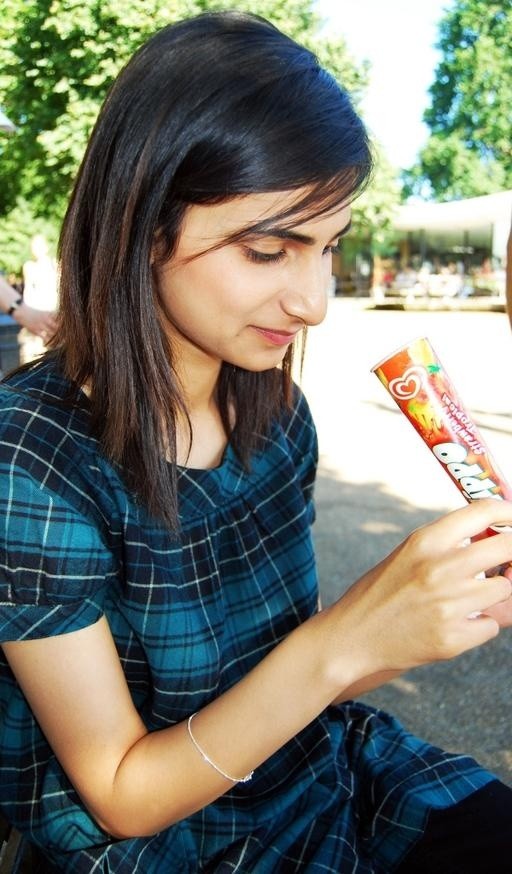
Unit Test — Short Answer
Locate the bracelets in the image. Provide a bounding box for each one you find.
[7,297,24,316]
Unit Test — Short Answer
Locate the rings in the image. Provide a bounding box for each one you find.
[42,330,48,336]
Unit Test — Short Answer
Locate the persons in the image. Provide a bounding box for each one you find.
[0,277,60,378]
[0,9,512,871]
[372,247,506,313]
